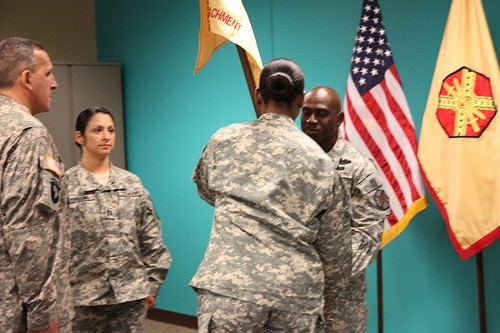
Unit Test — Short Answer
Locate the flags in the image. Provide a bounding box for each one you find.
[335,0,428,256]
[193,0,264,90]
[416,0,500,260]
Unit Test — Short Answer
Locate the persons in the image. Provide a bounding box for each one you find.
[301,86,389,333]
[188,58,336,333]
[66,107,170,333]
[0,36,69,333]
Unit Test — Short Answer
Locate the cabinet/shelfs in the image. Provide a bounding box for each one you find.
[33,60,125,169]
